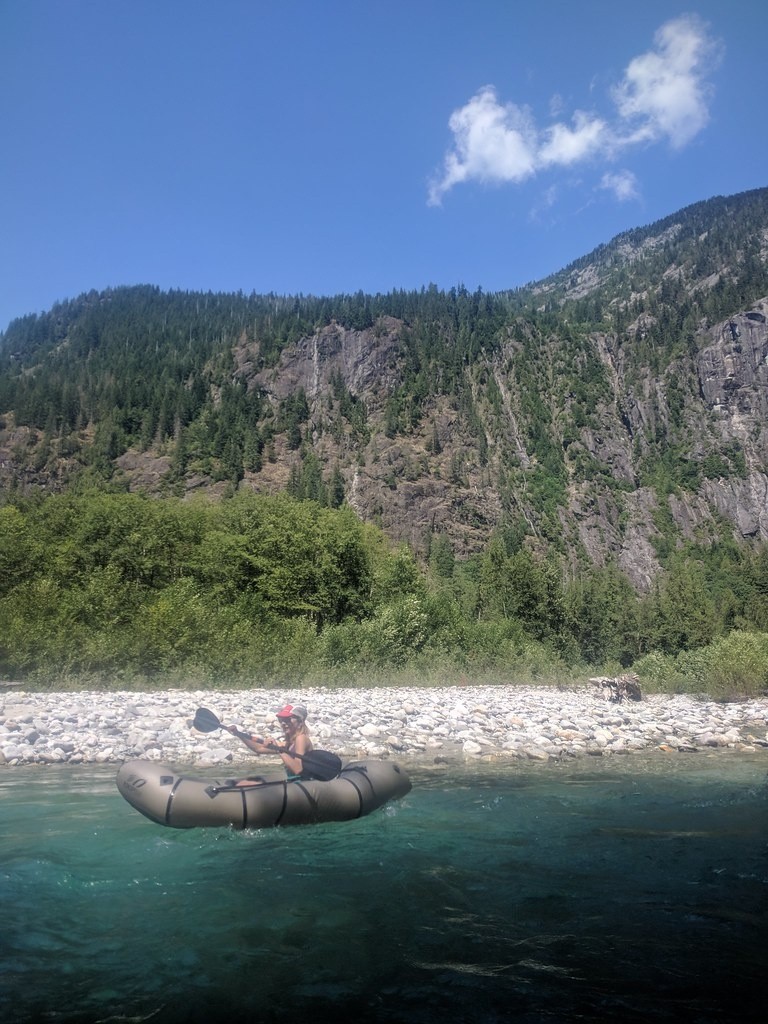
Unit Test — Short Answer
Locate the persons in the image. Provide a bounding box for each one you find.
[228,702,314,786]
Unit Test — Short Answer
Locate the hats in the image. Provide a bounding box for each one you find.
[276,703,308,721]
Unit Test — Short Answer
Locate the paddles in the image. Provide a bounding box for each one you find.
[193,708,342,781]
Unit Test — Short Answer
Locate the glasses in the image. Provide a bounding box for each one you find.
[278,717,291,723]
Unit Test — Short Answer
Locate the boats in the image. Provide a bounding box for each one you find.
[114,761,411,830]
